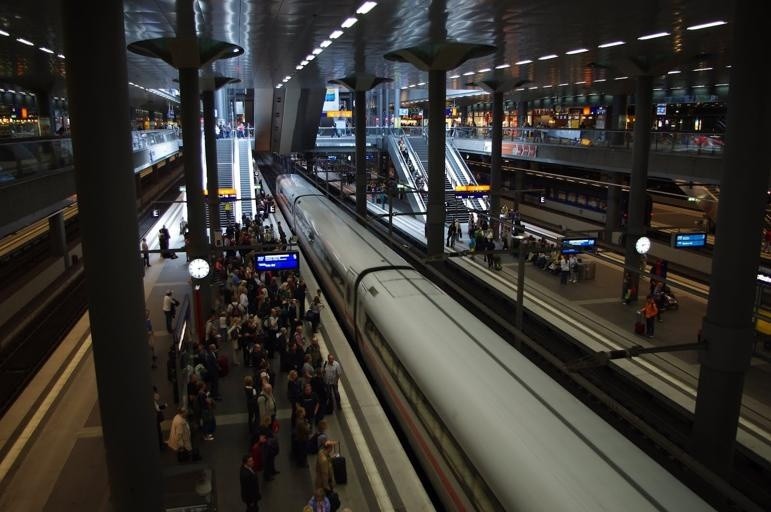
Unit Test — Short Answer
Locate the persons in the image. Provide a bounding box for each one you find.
[316,138,425,207]
[141,193,345,511]
[139,112,248,138]
[620,255,677,339]
[445,201,581,287]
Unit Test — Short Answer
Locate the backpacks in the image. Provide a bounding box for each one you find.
[231,303,241,317]
[307,432,323,454]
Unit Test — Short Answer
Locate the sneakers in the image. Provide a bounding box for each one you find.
[203,434,214,441]
[264,470,279,482]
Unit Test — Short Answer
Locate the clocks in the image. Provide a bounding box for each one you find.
[635,236,651,255]
[187,258,210,279]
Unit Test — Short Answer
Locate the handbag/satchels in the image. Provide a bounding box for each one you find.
[325,490,340,512]
[272,420,280,432]
[306,310,314,321]
[177,447,202,464]
[659,296,670,307]
[191,397,200,410]
[573,264,579,272]
[230,329,238,338]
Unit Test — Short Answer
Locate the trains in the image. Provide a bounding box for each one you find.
[0,127,184,268]
[276,173,771,512]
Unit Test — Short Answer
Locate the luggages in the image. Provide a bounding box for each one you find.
[217,355,230,377]
[331,441,347,484]
[324,385,333,415]
[635,311,645,334]
